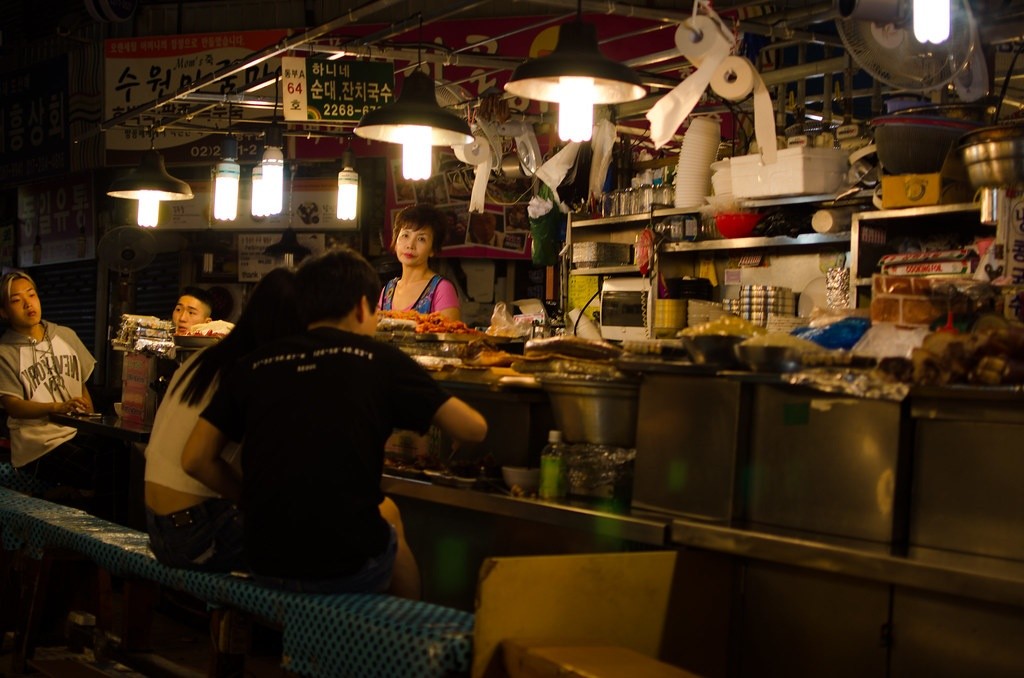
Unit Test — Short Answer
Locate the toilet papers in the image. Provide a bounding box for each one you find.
[645,15,730,150]
[449,136,493,214]
[711,57,778,167]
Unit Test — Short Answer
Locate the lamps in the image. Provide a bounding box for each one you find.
[503,0,647,142]
[353,0,475,180]
[186,163,231,272]
[265,166,311,266]
[106,131,196,229]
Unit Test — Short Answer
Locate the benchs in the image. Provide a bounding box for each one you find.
[0,462,468,678]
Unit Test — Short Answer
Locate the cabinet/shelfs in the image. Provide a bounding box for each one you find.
[562,204,1003,341]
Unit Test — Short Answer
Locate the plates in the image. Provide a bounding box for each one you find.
[171,334,219,348]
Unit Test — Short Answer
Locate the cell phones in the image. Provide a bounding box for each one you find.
[71,411,102,417]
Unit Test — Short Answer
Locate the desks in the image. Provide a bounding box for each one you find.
[49,413,155,525]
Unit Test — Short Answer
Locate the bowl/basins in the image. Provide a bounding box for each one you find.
[957,125,1024,191]
[673,115,732,209]
[715,212,764,239]
[502,465,539,494]
[536,377,641,447]
[687,299,722,327]
[415,333,475,359]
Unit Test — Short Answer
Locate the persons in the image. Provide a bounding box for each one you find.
[144,268,305,574]
[172,286,213,336]
[0,273,97,500]
[378,204,460,322]
[180,251,488,599]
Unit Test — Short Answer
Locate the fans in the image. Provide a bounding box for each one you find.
[97,226,157,286]
[834,0,1001,99]
[434,79,544,177]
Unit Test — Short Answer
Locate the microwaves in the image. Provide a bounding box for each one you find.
[600,276,650,342]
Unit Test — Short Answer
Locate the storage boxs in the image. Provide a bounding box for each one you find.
[120,354,162,421]
[905,374,1024,562]
[752,382,903,543]
[881,142,968,208]
[502,636,702,678]
[869,272,970,330]
[451,385,538,485]
[631,374,745,516]
[724,148,845,201]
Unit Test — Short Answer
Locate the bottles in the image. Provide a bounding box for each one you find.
[601,182,676,217]
[537,428,567,503]
